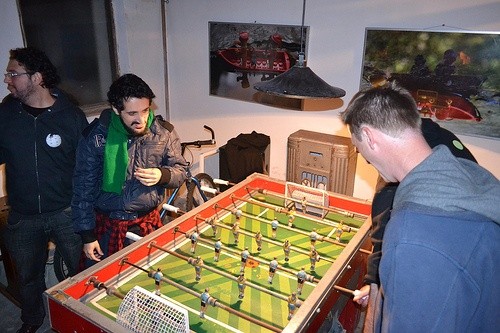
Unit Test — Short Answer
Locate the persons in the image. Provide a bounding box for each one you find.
[343,83,500,333]
[71,73,191,275]
[0,47,90,333]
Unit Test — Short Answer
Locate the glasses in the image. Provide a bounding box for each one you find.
[4,72,29,78]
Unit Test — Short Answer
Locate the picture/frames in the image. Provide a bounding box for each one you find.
[359,27,500,142]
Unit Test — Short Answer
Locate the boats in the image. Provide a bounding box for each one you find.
[406,85,481,121]
[218,48,290,73]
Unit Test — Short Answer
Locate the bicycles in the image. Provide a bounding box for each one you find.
[52,123,218,284]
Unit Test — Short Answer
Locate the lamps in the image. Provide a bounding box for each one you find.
[253,0,348,99]
